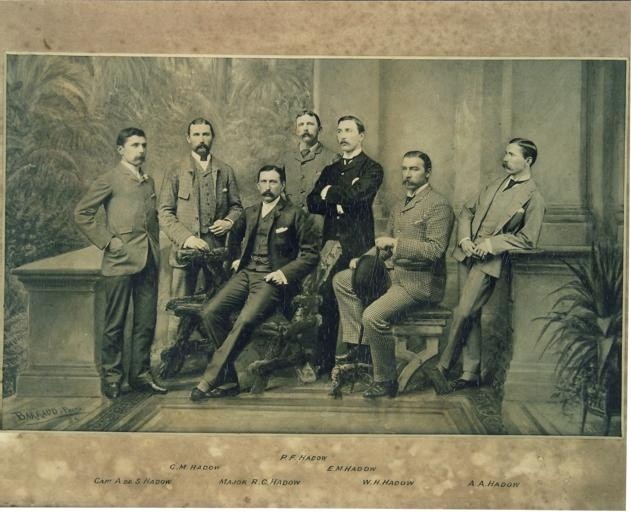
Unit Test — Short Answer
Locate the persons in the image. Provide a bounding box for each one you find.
[331,146,456,398]
[75,126,171,397]
[279,105,334,257]
[305,116,383,379]
[157,109,244,382]
[192,164,323,406]
[432,136,551,392]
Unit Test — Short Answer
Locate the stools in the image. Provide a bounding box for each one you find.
[389,300,453,392]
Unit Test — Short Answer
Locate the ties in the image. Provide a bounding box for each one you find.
[301,148,311,158]
[405,196,413,206]
[344,157,353,165]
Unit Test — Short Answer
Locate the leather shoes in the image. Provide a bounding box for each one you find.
[365,379,400,398]
[103,382,121,399]
[139,383,168,395]
[449,377,473,389]
[190,384,240,401]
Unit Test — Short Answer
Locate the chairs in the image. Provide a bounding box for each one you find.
[162,239,323,395]
